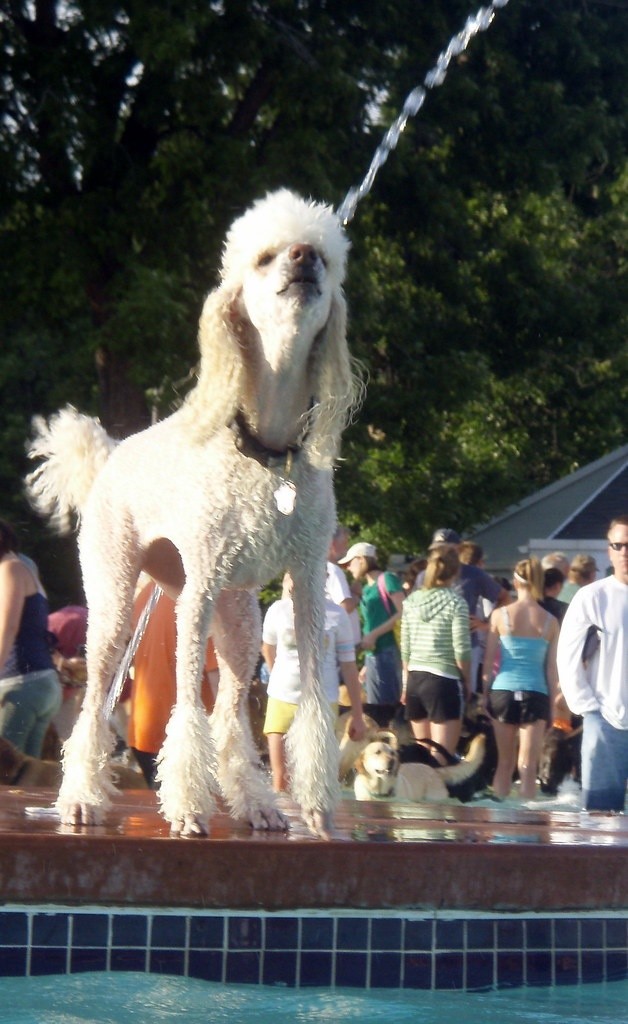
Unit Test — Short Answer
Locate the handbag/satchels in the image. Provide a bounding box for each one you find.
[378,571,401,653]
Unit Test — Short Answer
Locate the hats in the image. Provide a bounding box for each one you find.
[337,541,380,564]
[431,528,464,544]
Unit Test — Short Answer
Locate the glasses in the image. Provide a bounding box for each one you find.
[609,543,628,551]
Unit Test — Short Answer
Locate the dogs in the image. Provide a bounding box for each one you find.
[26,183,373,840]
[337,708,485,807]
[0,737,146,789]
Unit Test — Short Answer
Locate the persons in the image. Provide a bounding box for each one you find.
[0,522,598,804]
[556,511,628,811]
